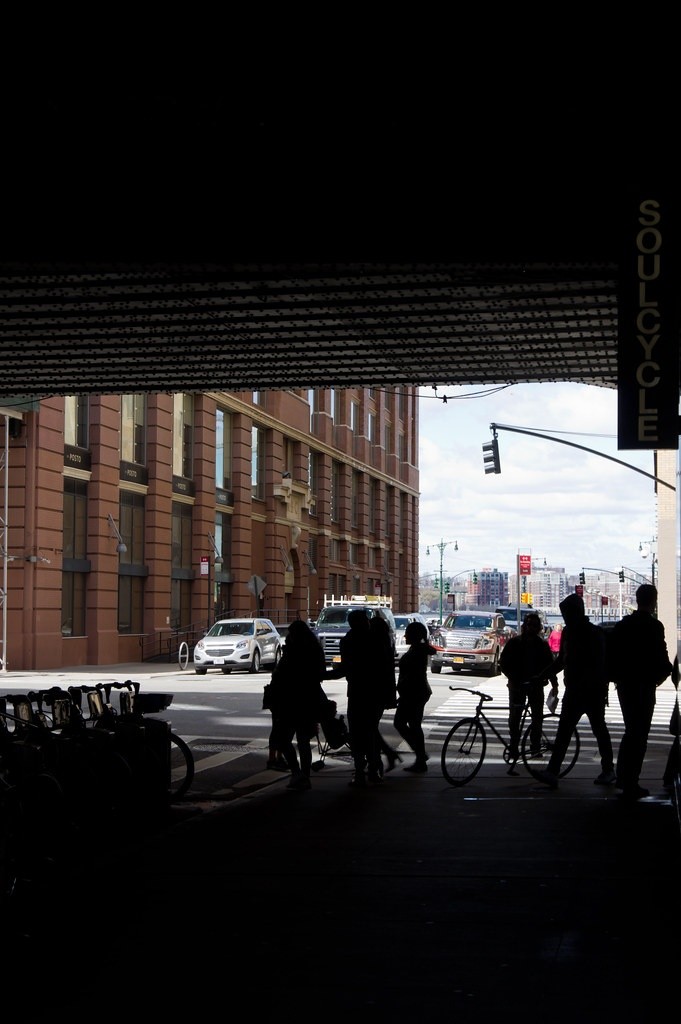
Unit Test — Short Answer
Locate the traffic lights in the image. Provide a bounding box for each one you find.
[579,572,585,584]
[619,571,624,583]
[521,593,532,604]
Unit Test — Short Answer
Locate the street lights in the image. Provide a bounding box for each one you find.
[517,558,547,634]
[638,541,654,585]
[426,540,459,627]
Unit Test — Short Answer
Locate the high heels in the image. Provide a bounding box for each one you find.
[367,760,384,777]
[385,752,403,773]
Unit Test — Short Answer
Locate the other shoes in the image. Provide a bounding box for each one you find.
[404,753,429,774]
[267,757,292,772]
[661,774,675,790]
[530,768,559,788]
[615,780,650,795]
[594,772,618,784]
[531,749,543,758]
[508,748,520,758]
[289,769,312,793]
[367,773,385,785]
[347,772,368,788]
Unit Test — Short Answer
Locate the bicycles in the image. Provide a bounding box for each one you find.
[441,685,580,786]
[0,681,195,909]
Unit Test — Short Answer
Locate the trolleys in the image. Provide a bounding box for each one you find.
[312,708,404,785]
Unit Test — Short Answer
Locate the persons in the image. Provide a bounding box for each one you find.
[531,592,617,788]
[663,657,681,787]
[394,621,436,773]
[549,623,563,660]
[606,584,674,796]
[265,610,396,791]
[500,614,558,758]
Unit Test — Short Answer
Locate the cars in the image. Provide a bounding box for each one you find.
[194,618,283,675]
[308,606,396,673]
[274,624,291,648]
[430,611,516,677]
[496,606,566,637]
[394,612,430,666]
